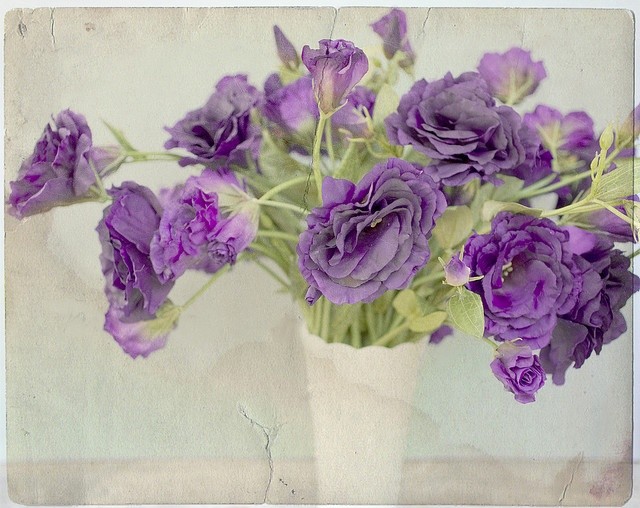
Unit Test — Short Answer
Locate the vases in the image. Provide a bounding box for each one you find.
[299,318,431,503]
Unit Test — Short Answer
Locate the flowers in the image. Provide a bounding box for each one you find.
[4,9,640,404]
